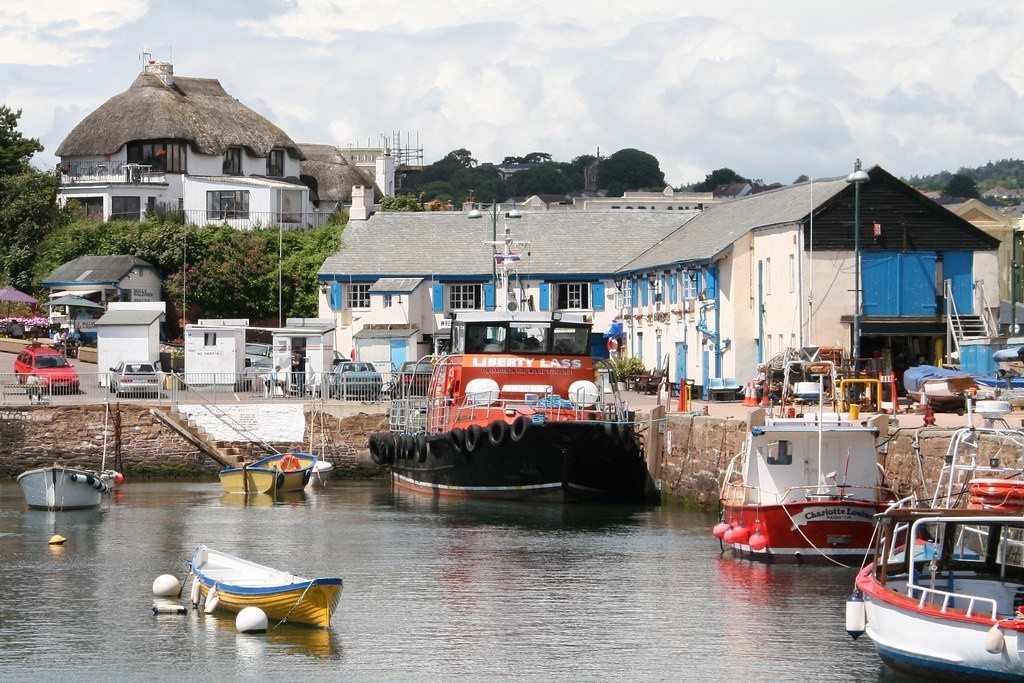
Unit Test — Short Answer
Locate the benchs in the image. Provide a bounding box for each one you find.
[199,561,239,579]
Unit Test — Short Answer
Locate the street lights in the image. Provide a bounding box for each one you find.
[847,158,871,406]
[469,199,522,300]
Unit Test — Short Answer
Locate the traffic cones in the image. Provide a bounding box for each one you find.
[918,384,929,410]
[885,372,906,414]
[747,381,758,406]
[761,380,770,405]
[741,382,750,405]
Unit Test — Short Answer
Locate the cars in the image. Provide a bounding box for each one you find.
[390,361,431,400]
[330,361,382,398]
[109,361,159,396]
[14,347,80,394]
[246,343,273,368]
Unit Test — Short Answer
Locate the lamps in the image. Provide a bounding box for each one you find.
[648,271,659,287]
[688,268,697,283]
[615,276,624,291]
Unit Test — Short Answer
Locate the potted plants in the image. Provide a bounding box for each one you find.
[699,290,706,301]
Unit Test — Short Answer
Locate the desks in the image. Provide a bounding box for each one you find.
[627,374,653,395]
[60,338,75,358]
[24,342,44,348]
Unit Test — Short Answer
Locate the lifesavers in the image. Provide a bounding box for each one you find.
[511,415,533,443]
[449,427,465,454]
[488,419,509,445]
[386,434,414,462]
[280,454,300,473]
[465,423,482,452]
[414,436,427,464]
[966,477,1024,513]
[608,335,622,355]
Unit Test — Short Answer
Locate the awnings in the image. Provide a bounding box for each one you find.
[49,290,101,297]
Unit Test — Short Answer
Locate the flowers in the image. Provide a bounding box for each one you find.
[685,307,695,313]
[635,314,642,319]
[647,312,652,323]
[660,312,669,321]
[671,309,682,314]
[624,314,631,319]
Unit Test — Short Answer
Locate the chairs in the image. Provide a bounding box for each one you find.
[707,377,741,403]
[48,344,79,358]
[627,367,665,395]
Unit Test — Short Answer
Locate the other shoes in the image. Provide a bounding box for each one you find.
[37,402,42,405]
[29,402,33,405]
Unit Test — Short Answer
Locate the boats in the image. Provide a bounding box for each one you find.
[368,225,660,504]
[308,462,333,487]
[17,467,105,509]
[904,367,981,403]
[190,543,342,629]
[712,361,911,565]
[219,453,317,495]
[845,394,1024,683]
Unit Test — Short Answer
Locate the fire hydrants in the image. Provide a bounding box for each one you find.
[922,404,936,427]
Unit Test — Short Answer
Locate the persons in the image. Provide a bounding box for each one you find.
[292,350,305,397]
[8,320,23,339]
[390,371,398,401]
[53,327,88,347]
[270,365,290,398]
[76,308,90,319]
[30,324,42,338]
[27,374,41,405]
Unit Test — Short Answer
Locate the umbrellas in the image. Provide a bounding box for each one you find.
[0,285,38,318]
[42,295,106,329]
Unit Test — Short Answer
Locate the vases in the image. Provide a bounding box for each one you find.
[665,316,669,321]
[677,313,681,319]
[628,318,631,323]
[638,320,641,324]
[688,312,695,318]
